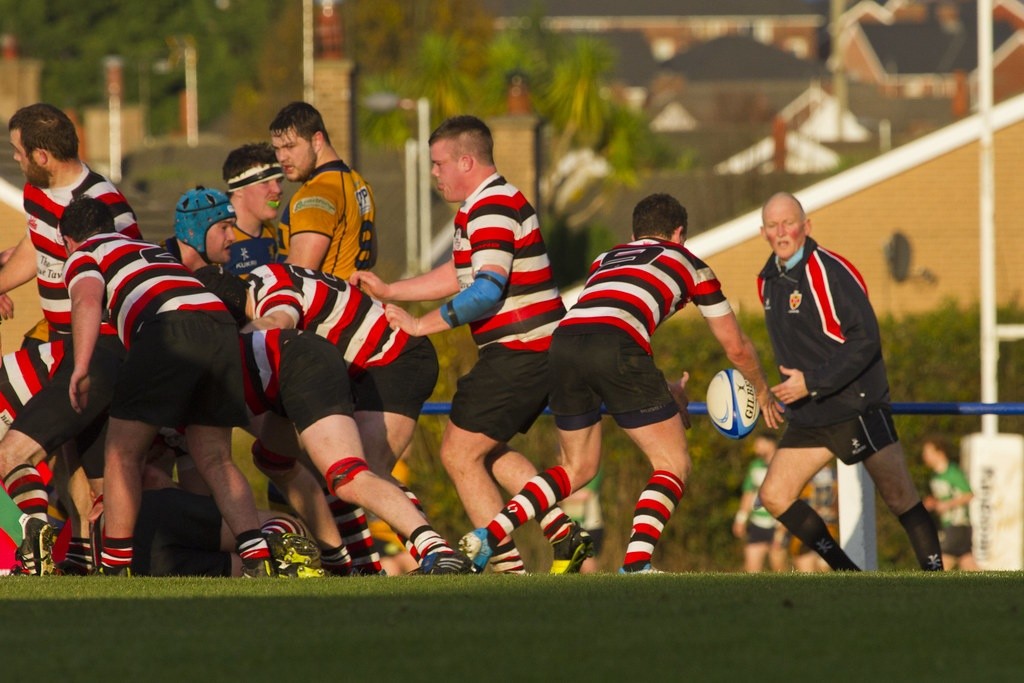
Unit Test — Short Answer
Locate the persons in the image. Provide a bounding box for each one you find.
[558,429,978,574]
[0,102,471,579]
[460,192,785,575]
[757,192,945,573]
[350,114,591,576]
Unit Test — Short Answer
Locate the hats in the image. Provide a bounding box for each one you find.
[173,184,239,266]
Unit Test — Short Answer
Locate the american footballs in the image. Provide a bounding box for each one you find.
[705,364,761,441]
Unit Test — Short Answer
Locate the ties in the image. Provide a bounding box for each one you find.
[772,266,791,318]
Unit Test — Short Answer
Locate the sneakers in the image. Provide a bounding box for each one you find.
[420,550,472,575]
[51,561,87,578]
[459,529,492,574]
[14,517,57,577]
[90,565,133,578]
[546,520,595,575]
[238,557,276,578]
